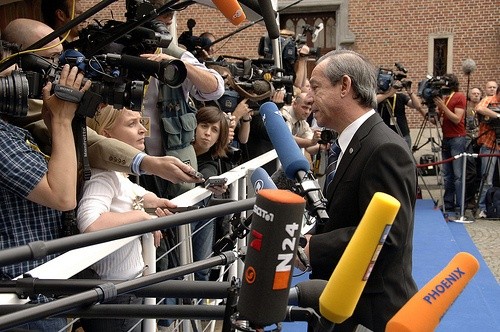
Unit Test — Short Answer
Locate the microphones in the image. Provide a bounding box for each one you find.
[211,0,339,332]
[462,59,476,74]
[385,252,480,332]
[316,192,401,332]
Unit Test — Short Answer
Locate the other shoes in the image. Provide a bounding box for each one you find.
[439,204,455,211]
[455,208,461,215]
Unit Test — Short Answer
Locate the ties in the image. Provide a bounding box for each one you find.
[326,139,341,194]
[292,121,300,135]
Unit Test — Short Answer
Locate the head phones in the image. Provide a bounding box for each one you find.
[468,93,483,101]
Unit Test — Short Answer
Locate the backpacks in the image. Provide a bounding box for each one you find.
[486,187,500,218]
[420,154,440,176]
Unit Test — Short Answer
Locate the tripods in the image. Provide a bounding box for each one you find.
[412,108,448,218]
[473,132,500,217]
[381,99,437,207]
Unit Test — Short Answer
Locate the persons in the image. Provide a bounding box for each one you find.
[418,73,466,216]
[465,88,482,210]
[376,78,421,150]
[475,85,500,220]
[196,31,218,63]
[485,81,497,96]
[293,51,420,332]
[0,19,331,332]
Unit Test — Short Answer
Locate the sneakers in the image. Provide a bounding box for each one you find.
[479,210,487,219]
